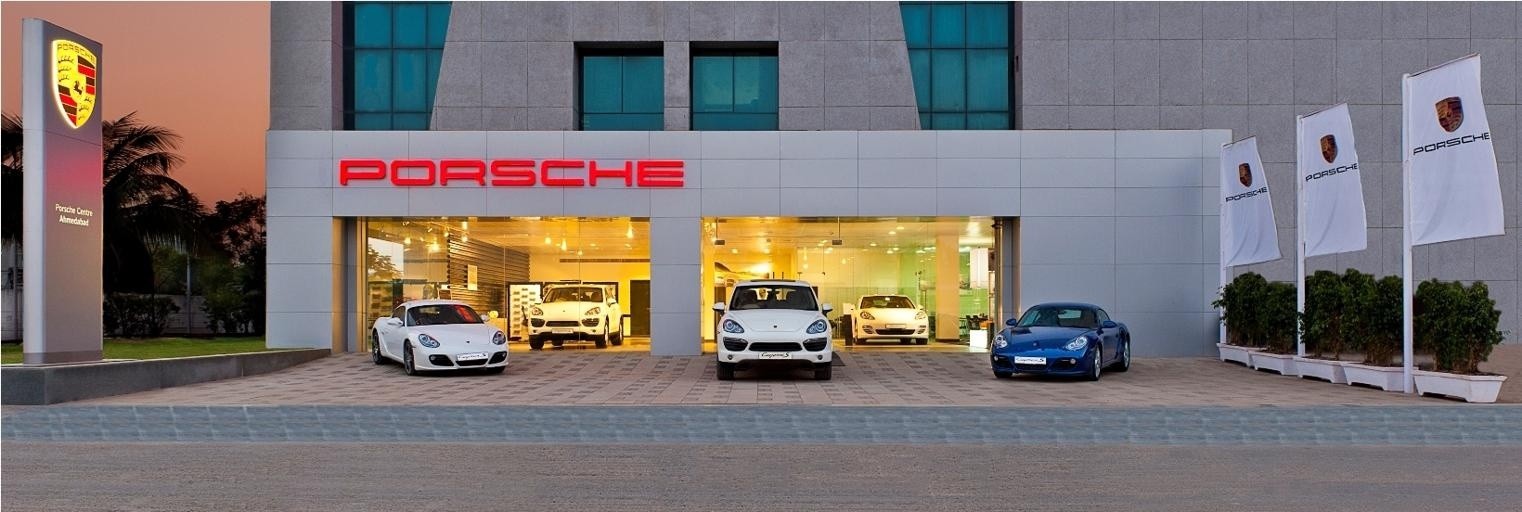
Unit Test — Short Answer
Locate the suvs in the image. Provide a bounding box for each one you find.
[714,279,836,381]
[526,285,626,350]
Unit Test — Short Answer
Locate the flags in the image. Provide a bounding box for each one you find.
[1298,103,1370,257]
[1407,55,1505,247]
[1222,136,1282,270]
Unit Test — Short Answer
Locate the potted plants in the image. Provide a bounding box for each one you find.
[1410,273,1514,404]
[1211,270,1267,368]
[1337,264,1421,397]
[1291,263,1349,387]
[1248,277,1303,378]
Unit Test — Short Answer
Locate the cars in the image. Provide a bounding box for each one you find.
[371,298,509,378]
[991,302,1133,382]
[839,293,931,348]
